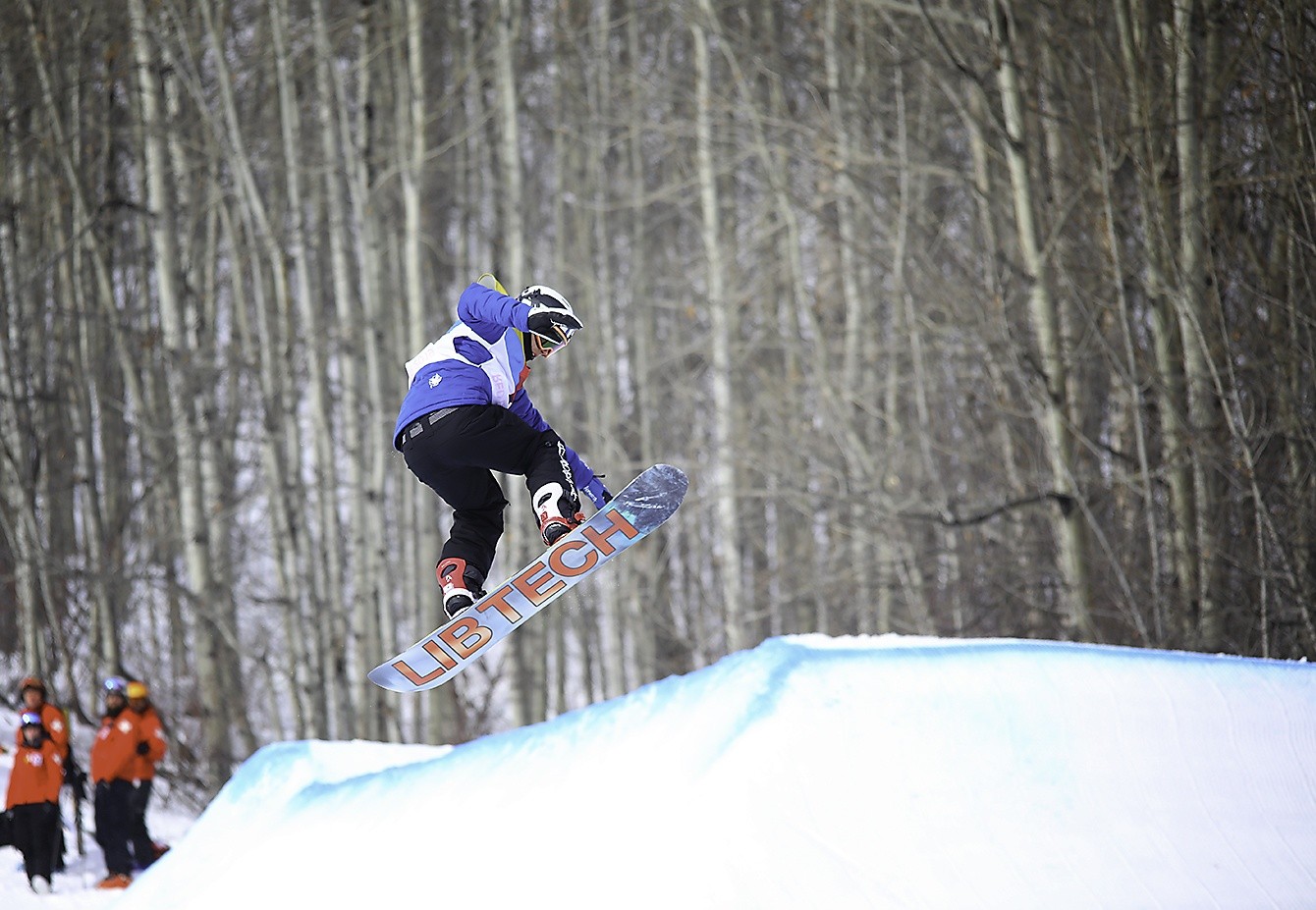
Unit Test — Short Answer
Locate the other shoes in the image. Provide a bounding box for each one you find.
[435,558,484,619]
[532,483,581,547]
[98,872,131,888]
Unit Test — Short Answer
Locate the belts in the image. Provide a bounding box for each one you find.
[402,405,459,445]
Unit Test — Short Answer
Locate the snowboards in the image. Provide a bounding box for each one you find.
[367,463,688,693]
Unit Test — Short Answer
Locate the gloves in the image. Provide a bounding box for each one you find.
[139,739,151,756]
[528,306,583,343]
[583,476,614,511]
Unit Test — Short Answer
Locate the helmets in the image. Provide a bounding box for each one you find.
[19,713,40,728]
[515,285,576,342]
[103,676,128,695]
[127,684,146,699]
[20,677,46,698]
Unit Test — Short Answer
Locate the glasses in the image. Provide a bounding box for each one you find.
[536,325,568,358]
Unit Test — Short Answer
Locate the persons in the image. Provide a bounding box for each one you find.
[90,675,171,888]
[393,274,613,623]
[7,677,69,893]
